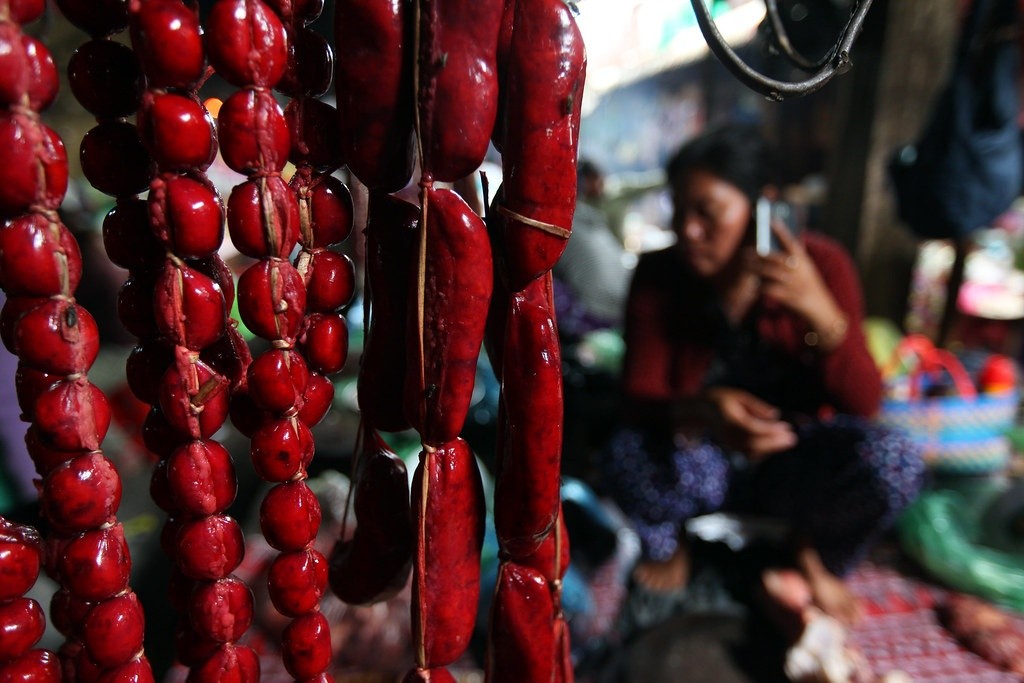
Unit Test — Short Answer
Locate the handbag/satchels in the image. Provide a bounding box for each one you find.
[885,53,1024,241]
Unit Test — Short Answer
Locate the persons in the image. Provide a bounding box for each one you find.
[561,126,924,640]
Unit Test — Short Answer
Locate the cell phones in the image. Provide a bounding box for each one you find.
[757,196,797,258]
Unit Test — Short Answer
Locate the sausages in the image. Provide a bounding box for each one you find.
[0,0,587,683]
